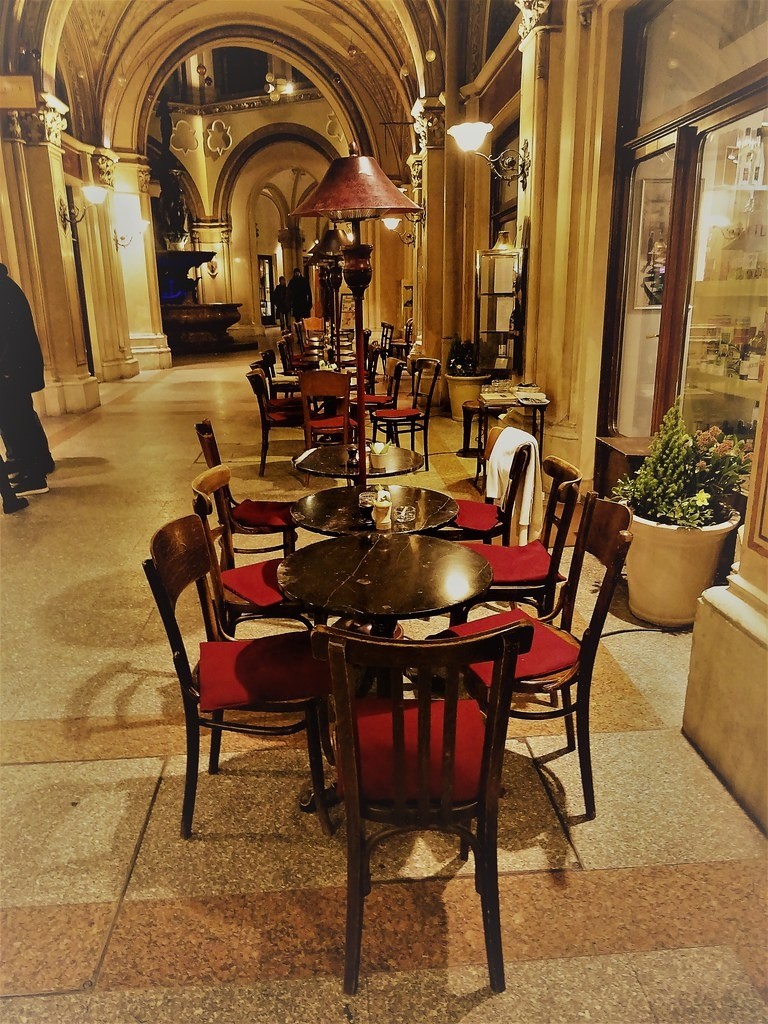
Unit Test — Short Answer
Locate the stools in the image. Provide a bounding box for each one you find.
[461,398,495,457]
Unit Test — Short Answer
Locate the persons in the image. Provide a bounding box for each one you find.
[273,276,291,335]
[0,263,55,497]
[288,268,313,322]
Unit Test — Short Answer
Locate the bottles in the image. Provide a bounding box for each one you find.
[735,126,764,183]
[709,418,758,458]
[699,315,767,382]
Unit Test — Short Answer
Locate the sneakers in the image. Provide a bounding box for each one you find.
[11,472,28,483]
[12,479,50,496]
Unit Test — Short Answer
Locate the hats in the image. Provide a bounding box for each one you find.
[294,268,301,272]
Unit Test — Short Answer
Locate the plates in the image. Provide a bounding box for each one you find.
[517,398,550,405]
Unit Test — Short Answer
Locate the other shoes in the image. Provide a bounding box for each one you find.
[282,332,285,336]
[5,497,29,513]
[5,452,13,460]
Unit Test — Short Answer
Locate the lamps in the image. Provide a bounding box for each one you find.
[59,184,107,232]
[114,218,150,252]
[446,122,530,192]
[382,216,414,248]
[399,186,427,229]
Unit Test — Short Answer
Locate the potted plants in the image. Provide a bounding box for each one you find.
[443,338,492,423]
[624,397,748,630]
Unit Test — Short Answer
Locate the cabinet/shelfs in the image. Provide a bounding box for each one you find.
[685,182,768,403]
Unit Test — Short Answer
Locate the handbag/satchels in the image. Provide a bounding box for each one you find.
[276,307,280,319]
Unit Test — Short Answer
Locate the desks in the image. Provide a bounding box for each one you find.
[304,349,354,355]
[299,355,357,366]
[292,377,370,410]
[310,337,349,341]
[474,395,549,496]
[312,331,350,338]
[291,439,424,482]
[276,534,493,815]
[308,342,353,350]
[295,367,364,376]
[290,485,459,535]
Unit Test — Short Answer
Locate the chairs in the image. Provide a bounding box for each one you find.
[143,320,632,998]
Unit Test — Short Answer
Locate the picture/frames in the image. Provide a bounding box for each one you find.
[632,178,705,311]
